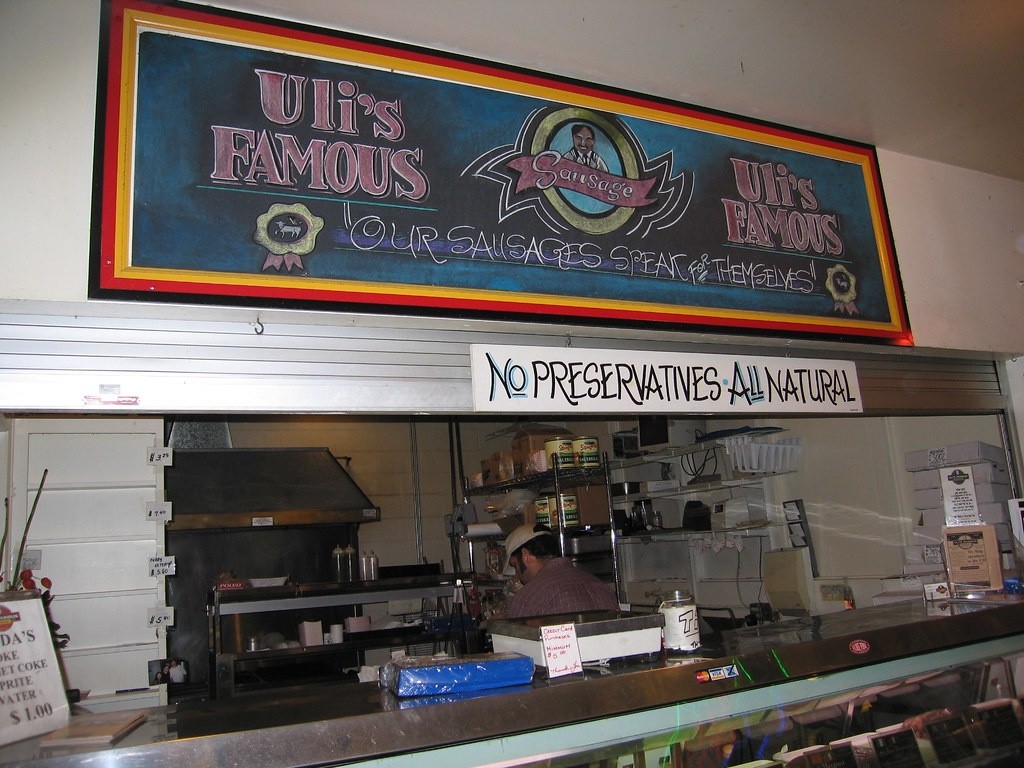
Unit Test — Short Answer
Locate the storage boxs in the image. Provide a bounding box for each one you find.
[511,426,577,464]
[640,479,680,492]
[871,440,1013,606]
[378,650,536,697]
[480,459,499,485]
[490,452,515,482]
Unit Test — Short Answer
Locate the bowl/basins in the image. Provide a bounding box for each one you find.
[250,577,288,587]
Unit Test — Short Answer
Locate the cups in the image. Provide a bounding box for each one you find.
[330,624,344,643]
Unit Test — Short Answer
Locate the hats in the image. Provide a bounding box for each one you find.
[502,522,553,576]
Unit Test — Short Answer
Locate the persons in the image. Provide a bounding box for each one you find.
[501,524,616,620]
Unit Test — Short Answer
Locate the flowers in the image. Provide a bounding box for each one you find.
[0,569,70,649]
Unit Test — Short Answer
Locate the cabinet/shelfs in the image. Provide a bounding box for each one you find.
[464,451,622,601]
[608,437,777,608]
[207,570,476,701]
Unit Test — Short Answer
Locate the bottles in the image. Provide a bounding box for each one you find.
[485,541,502,576]
[369,550,379,581]
[248,636,259,651]
[483,589,506,614]
[660,589,702,658]
[842,576,855,610]
[358,551,370,581]
[344,544,357,583]
[331,544,345,583]
[653,510,663,530]
[634,499,653,530]
